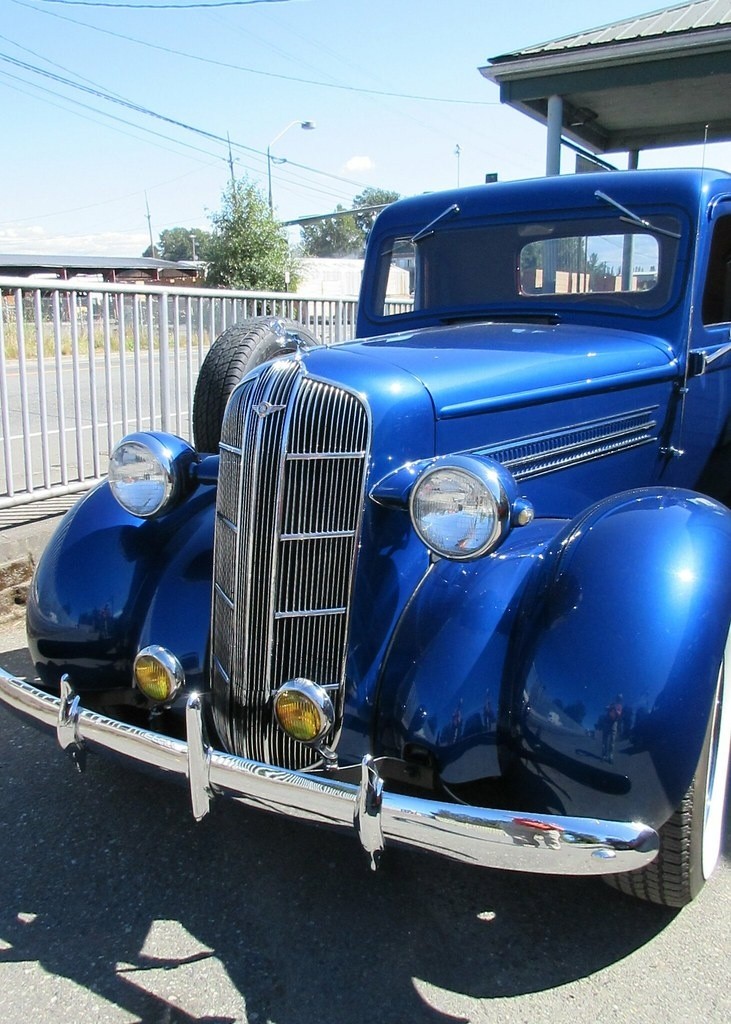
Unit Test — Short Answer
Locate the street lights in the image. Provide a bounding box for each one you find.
[267,120,318,258]
[454,142,461,189]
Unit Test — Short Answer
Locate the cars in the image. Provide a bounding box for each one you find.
[0,165,730,909]
